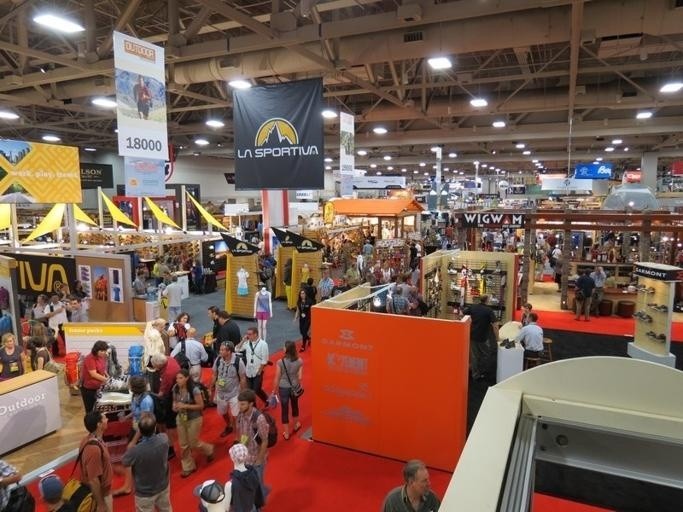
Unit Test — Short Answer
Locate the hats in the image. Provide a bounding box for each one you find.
[194,479,225,504]
[37,474,63,498]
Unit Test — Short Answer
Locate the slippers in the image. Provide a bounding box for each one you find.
[294,423,302,432]
[283,431,290,440]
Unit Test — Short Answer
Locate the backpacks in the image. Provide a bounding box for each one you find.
[252,411,277,448]
[192,382,212,407]
[150,392,166,425]
[174,341,189,369]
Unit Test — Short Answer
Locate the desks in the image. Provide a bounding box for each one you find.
[0,369,61,458]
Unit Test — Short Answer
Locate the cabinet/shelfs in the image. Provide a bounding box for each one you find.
[633,286,669,344]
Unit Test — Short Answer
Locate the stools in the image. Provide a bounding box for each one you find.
[540,338,553,362]
[524,357,541,370]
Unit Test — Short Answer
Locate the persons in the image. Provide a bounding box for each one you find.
[317,196,611,380]
[0,196,311,512]
[381,459,441,511]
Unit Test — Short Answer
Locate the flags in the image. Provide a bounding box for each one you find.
[111,30,170,161]
[228,77,325,192]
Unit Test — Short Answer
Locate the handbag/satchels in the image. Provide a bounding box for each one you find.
[290,385,304,400]
[7,487,35,511]
[200,345,215,368]
[62,479,96,511]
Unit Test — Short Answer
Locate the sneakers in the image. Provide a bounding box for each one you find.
[181,465,197,477]
[208,446,216,463]
[221,426,232,437]
